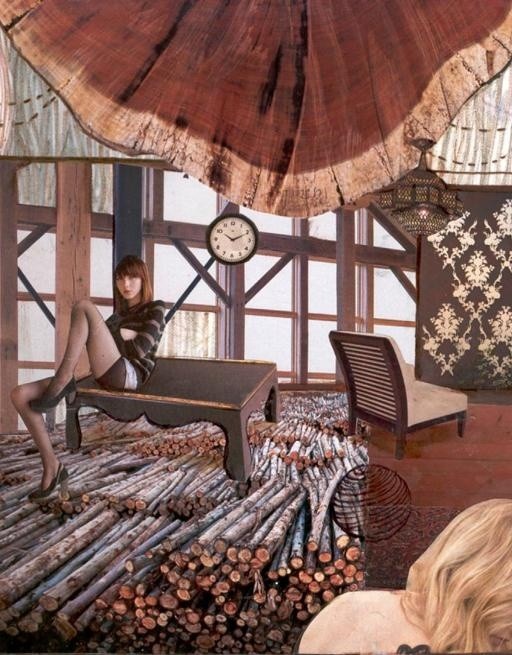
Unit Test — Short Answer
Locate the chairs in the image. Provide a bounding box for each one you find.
[329,333,467,455]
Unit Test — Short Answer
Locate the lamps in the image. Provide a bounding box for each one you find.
[379,138,464,240]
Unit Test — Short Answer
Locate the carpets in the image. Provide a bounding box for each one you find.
[361,505,465,591]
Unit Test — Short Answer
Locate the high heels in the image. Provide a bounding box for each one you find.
[28,463,71,503]
[30,373,79,412]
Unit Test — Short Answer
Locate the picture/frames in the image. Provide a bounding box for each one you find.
[418,186,512,390]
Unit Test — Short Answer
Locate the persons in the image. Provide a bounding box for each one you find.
[400,497,511,654]
[9,256,166,508]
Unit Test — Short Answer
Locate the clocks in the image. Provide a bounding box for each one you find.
[206,213,260,265]
